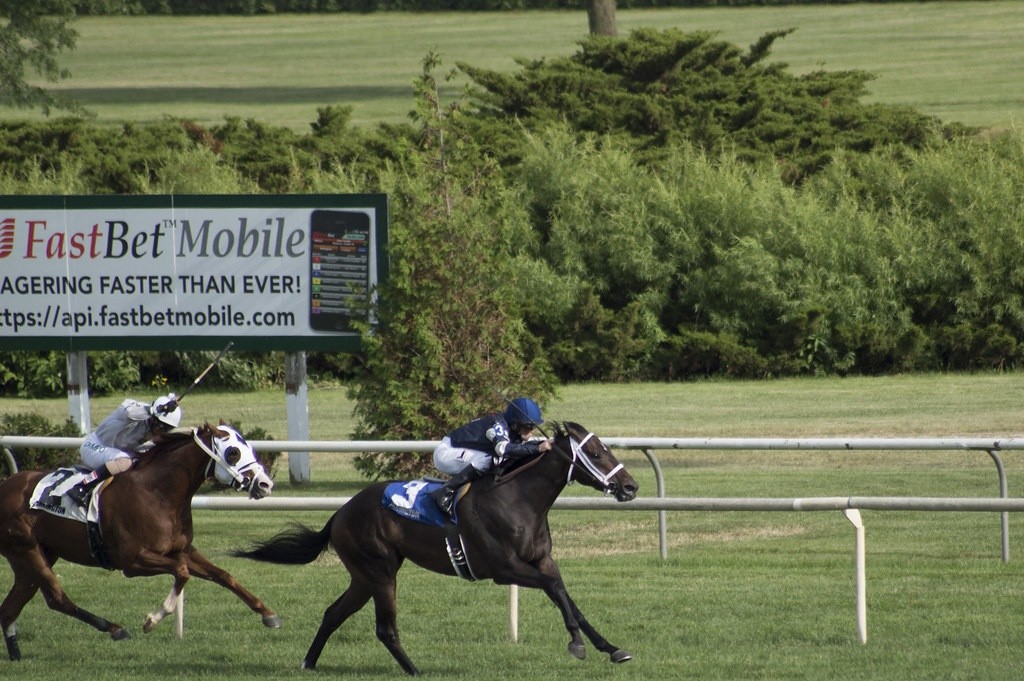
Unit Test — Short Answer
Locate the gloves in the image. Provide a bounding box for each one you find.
[158,400,179,413]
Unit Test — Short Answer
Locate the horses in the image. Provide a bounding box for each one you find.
[221,420,639,675]
[0,419,280,663]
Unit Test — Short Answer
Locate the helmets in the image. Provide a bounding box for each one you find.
[505,398,543,424]
[152,393,181,428]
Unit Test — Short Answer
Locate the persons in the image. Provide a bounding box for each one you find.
[431,397,553,518]
[66,397,182,507]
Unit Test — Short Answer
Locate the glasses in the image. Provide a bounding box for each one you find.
[517,424,536,430]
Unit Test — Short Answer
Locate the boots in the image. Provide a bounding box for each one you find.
[67,463,112,507]
[431,463,481,518]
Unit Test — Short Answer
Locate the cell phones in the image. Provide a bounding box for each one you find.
[308,211,371,333]
